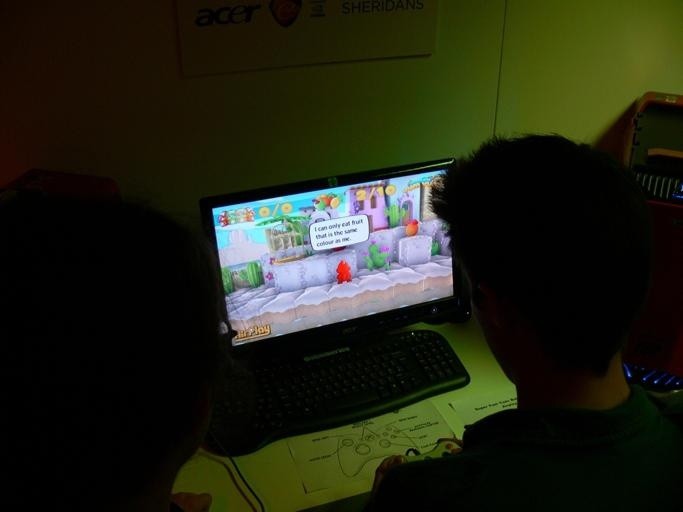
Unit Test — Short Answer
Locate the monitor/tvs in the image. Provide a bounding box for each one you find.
[198,157,466,360]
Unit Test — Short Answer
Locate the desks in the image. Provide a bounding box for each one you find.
[170,297,522,512]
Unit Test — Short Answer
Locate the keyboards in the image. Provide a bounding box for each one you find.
[195,330,471,458]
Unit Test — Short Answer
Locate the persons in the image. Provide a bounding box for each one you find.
[0,188,234,511]
[360,134,683,511]
